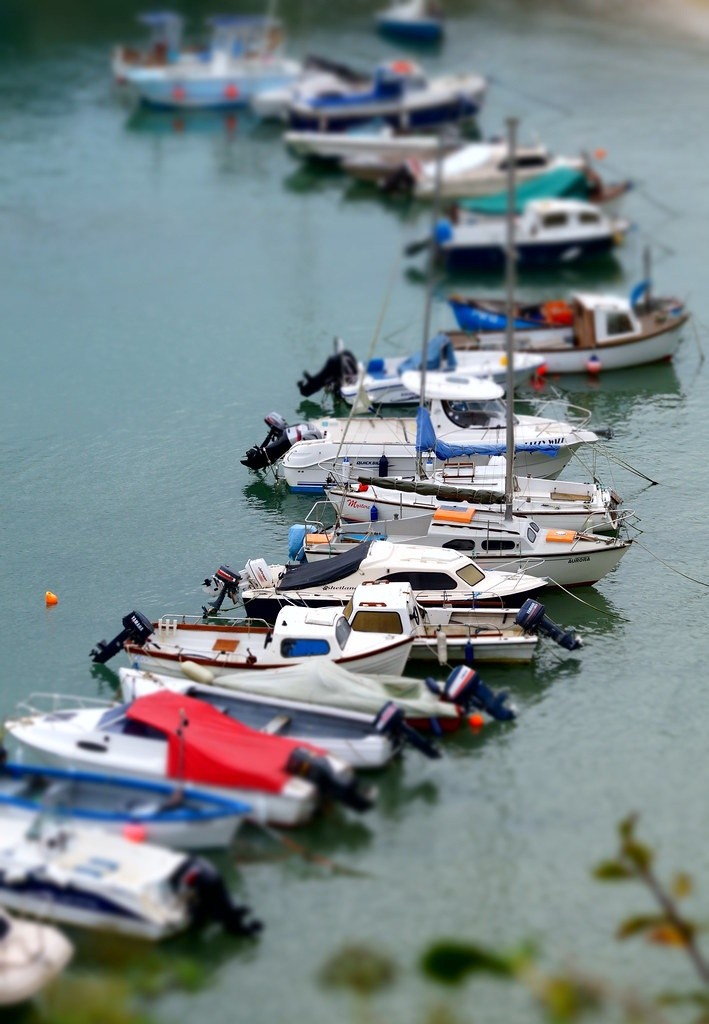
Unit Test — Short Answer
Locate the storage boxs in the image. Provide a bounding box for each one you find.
[245,558,272,588]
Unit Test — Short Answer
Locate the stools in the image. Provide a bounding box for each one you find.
[211,640,239,654]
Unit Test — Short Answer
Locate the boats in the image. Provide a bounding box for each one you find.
[1,285,694,1024]
[456,164,602,221]
[430,195,635,273]
[375,1,447,56]
[246,45,489,131]
[108,10,306,115]
[413,141,632,206]
[278,124,472,191]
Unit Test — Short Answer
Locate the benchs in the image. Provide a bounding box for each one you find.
[551,492,591,502]
